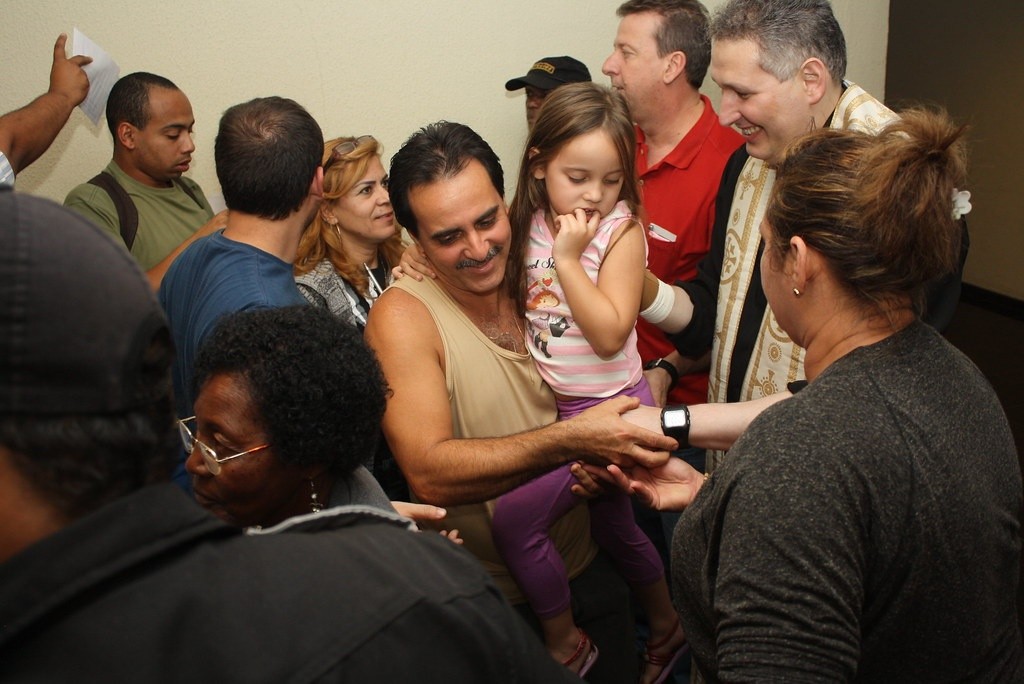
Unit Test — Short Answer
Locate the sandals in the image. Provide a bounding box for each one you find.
[636,614,689,684]
[559,627,599,679]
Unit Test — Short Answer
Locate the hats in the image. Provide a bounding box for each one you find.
[0,182,186,460]
[505,56,593,94]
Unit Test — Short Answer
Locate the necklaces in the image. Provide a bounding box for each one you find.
[487,302,526,353]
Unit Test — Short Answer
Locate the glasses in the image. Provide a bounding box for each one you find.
[322,135,375,174]
[176,414,272,476]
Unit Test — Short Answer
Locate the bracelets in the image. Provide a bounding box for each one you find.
[644,358,679,392]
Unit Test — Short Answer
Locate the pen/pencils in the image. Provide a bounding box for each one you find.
[364,263,383,294]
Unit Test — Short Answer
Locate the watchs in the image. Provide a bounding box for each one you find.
[659,404,691,448]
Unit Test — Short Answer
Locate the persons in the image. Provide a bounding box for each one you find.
[158,96,327,499]
[391,83,692,684]
[0,33,92,191]
[619,0,970,479]
[506,56,592,129]
[363,122,680,684]
[581,110,1024,684]
[62,72,230,302]
[294,134,406,332]
[600,0,749,557]
[172,300,414,535]
[0,188,579,684]
